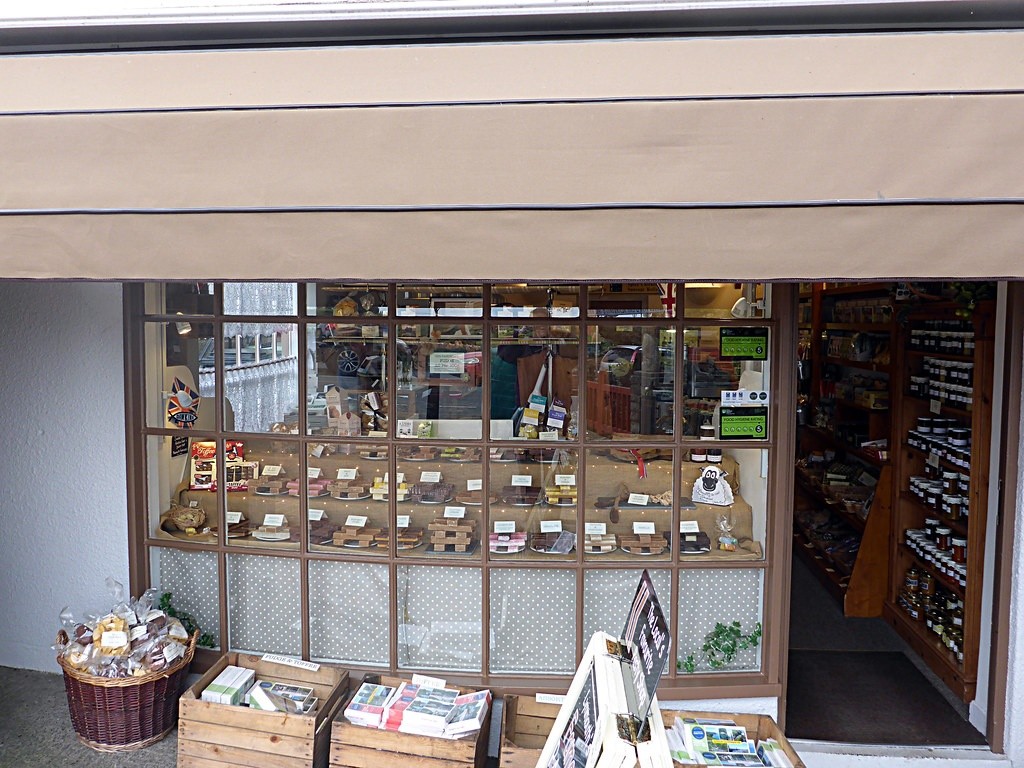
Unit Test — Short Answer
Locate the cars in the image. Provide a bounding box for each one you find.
[459,352,483,386]
[599,345,677,386]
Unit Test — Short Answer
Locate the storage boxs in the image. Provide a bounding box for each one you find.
[176,650,495,768]
[499,694,807,768]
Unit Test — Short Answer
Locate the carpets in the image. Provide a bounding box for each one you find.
[785,647,986,745]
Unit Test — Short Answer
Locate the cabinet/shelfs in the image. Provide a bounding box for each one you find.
[802,281,996,701]
[347,384,430,431]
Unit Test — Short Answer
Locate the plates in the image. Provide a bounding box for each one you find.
[418,497,453,504]
[490,546,525,553]
[449,459,478,462]
[668,545,711,553]
[255,491,288,495]
[490,459,517,462]
[503,499,543,506]
[343,541,377,547]
[386,541,422,550]
[401,455,429,461]
[545,500,576,506]
[530,546,562,554]
[256,537,287,541]
[531,458,561,464]
[212,530,252,537]
[334,494,371,500]
[358,455,401,460]
[372,496,411,501]
[574,546,617,554]
[292,492,329,497]
[319,540,332,544]
[461,498,497,505]
[621,547,664,555]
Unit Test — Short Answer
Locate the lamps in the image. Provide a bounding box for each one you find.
[167,312,193,334]
[729,298,765,318]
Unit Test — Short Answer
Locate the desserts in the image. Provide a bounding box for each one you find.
[210,424,710,555]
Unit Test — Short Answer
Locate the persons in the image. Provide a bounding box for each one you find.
[336,311,412,413]
[497,307,579,438]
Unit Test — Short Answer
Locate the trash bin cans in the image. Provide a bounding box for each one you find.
[356,355,388,418]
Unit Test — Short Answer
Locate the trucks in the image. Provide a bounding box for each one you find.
[316,325,411,377]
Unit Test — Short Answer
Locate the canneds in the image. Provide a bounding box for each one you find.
[897,328,976,663]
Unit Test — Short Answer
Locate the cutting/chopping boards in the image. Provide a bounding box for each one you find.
[603,497,696,509]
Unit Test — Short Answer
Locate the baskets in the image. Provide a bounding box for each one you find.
[56,627,201,753]
[171,508,205,530]
